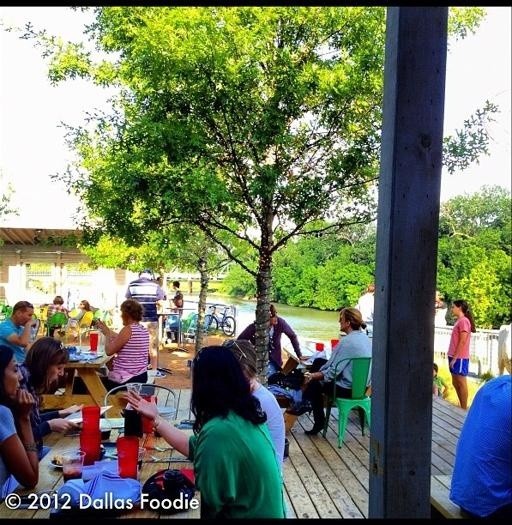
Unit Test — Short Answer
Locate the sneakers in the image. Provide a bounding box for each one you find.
[154,370,166,379]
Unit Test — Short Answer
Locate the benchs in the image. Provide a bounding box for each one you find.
[429,471,467,524]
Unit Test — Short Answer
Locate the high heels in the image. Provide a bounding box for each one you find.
[305,417,327,435]
[285,401,313,417]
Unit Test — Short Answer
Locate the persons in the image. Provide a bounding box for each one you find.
[432,364,448,400]
[450,374,512,524]
[447,298,476,411]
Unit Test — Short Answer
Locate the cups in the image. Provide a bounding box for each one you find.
[158,406,176,426]
[62,450,86,483]
[316,342,325,351]
[89,332,99,351]
[77,383,156,481]
[331,339,340,348]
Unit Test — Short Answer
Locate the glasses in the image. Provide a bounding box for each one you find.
[222,339,247,359]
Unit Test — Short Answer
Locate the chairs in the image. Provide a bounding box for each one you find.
[0,291,372,520]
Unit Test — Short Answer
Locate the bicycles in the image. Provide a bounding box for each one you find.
[205,304,237,339]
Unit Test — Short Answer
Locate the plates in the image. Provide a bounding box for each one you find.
[50,457,64,470]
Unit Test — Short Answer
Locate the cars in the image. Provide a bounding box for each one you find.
[126,281,167,321]
